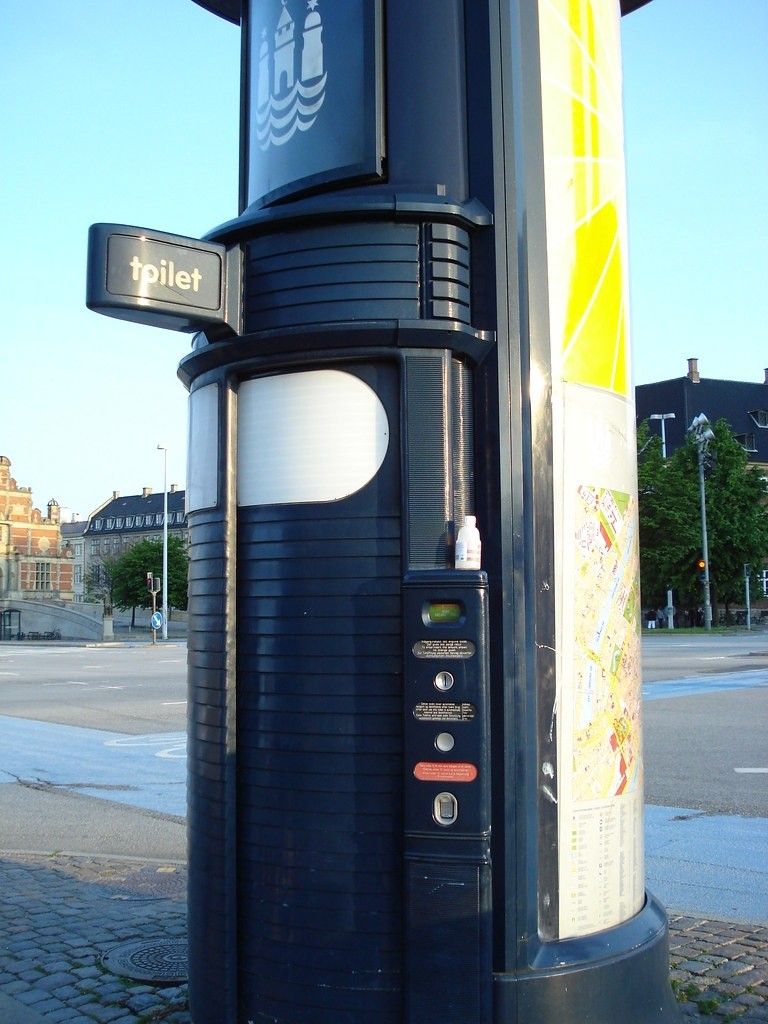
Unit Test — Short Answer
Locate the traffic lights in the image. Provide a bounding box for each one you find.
[147,578,153,592]
[744,564,751,577]
[698,560,705,580]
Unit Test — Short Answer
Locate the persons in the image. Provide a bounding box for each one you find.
[656,609,665,628]
[646,609,656,629]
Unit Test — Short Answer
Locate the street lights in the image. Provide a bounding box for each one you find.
[650,412,676,469]
[690,410,715,634]
[155,443,169,639]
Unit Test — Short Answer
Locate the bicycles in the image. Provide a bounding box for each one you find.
[719,615,768,626]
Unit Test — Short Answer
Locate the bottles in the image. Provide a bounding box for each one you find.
[454,517,482,571]
